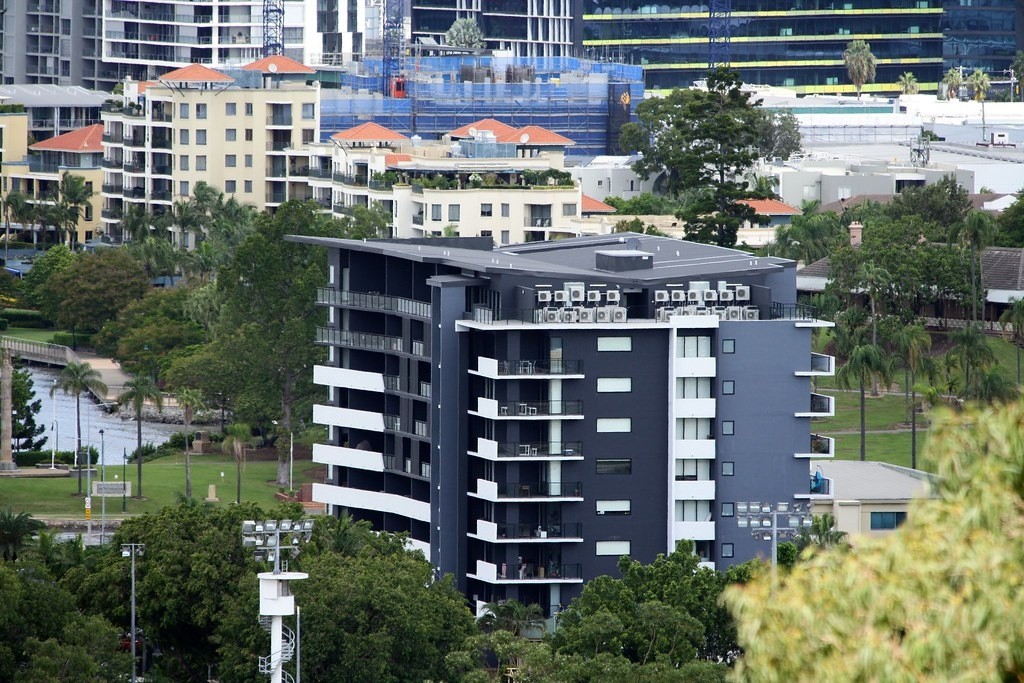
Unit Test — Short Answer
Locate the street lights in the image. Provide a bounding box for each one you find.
[50,420,59,452]
[270,418,293,495]
[48,378,59,469]
[122,445,128,512]
[118,542,147,683]
[240,516,317,683]
[85,401,120,544]
[97,429,105,481]
[734,500,816,599]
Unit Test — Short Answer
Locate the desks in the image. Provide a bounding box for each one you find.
[514,360,530,374]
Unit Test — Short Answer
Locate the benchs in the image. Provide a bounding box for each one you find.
[532,447,537,456]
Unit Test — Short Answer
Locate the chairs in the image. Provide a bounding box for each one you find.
[502,359,536,376]
[518,564,527,580]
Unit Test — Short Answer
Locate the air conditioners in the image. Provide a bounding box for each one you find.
[537,284,759,324]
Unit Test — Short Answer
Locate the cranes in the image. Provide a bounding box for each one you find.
[259,1,288,59]
[707,0,731,79]
[381,1,406,96]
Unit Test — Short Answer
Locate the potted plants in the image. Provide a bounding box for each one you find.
[69,464,98,478]
[35,458,68,470]
